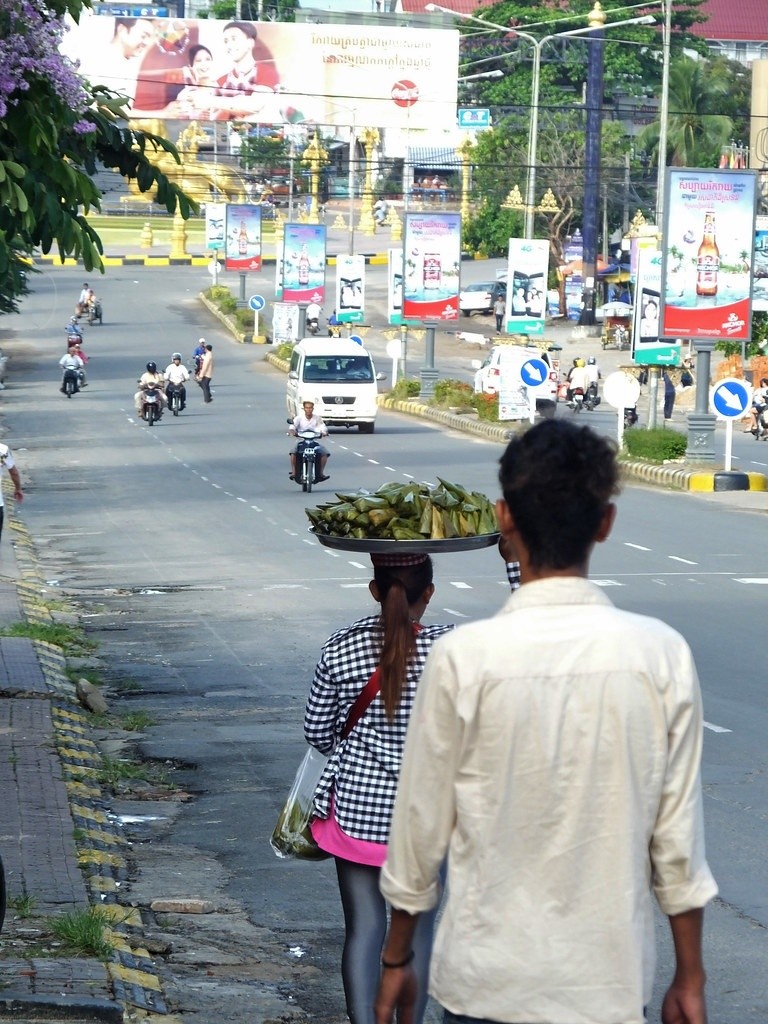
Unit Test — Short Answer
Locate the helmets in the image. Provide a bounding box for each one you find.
[587,357,596,364]
[147,362,156,371]
[574,357,581,367]
[173,354,181,360]
[577,360,585,366]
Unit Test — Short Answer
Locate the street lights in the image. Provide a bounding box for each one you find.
[425,2,659,346]
[348,69,502,251]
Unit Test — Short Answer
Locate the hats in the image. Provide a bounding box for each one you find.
[370,552,428,567]
[199,338,205,343]
[76,344,79,347]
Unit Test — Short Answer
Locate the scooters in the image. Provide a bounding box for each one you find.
[65,328,84,349]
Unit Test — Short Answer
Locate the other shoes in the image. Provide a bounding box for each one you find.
[315,475,330,482]
[290,476,295,480]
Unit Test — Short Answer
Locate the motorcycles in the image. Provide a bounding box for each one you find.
[76,300,102,326]
[565,375,636,430]
[307,319,318,336]
[60,357,89,399]
[328,319,341,338]
[599,310,632,351]
[162,370,191,415]
[137,379,165,426]
[752,405,768,441]
[286,418,330,493]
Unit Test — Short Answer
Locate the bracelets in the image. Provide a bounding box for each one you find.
[383,951,414,968]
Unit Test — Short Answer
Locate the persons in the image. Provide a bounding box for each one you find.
[493,296,506,334]
[304,536,522,1024]
[163,353,190,412]
[416,175,448,200]
[745,379,768,434]
[91,18,281,120]
[76,283,96,318]
[134,361,168,417]
[374,419,719,1024]
[305,302,322,331]
[328,310,343,338]
[260,199,276,207]
[641,301,658,337]
[513,287,542,318]
[194,338,213,403]
[0,443,24,543]
[609,287,629,302]
[566,357,601,405]
[373,196,388,228]
[347,358,371,379]
[59,316,88,393]
[607,315,622,329]
[290,402,331,483]
[663,354,694,419]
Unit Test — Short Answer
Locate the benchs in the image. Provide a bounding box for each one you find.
[608,317,630,327]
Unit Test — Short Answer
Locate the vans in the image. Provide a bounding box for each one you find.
[287,338,387,433]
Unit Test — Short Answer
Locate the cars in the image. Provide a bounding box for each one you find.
[196,127,216,154]
[256,174,301,197]
[460,281,508,317]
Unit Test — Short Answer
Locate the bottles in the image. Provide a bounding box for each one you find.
[298,243,310,285]
[696,206,719,296]
[239,220,248,254]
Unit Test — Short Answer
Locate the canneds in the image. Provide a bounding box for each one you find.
[423,253,441,290]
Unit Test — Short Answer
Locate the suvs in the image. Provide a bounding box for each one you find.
[471,347,559,418]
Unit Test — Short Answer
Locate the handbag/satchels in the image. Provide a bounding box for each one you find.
[269,745,335,861]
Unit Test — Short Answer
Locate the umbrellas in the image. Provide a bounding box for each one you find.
[567,259,632,288]
